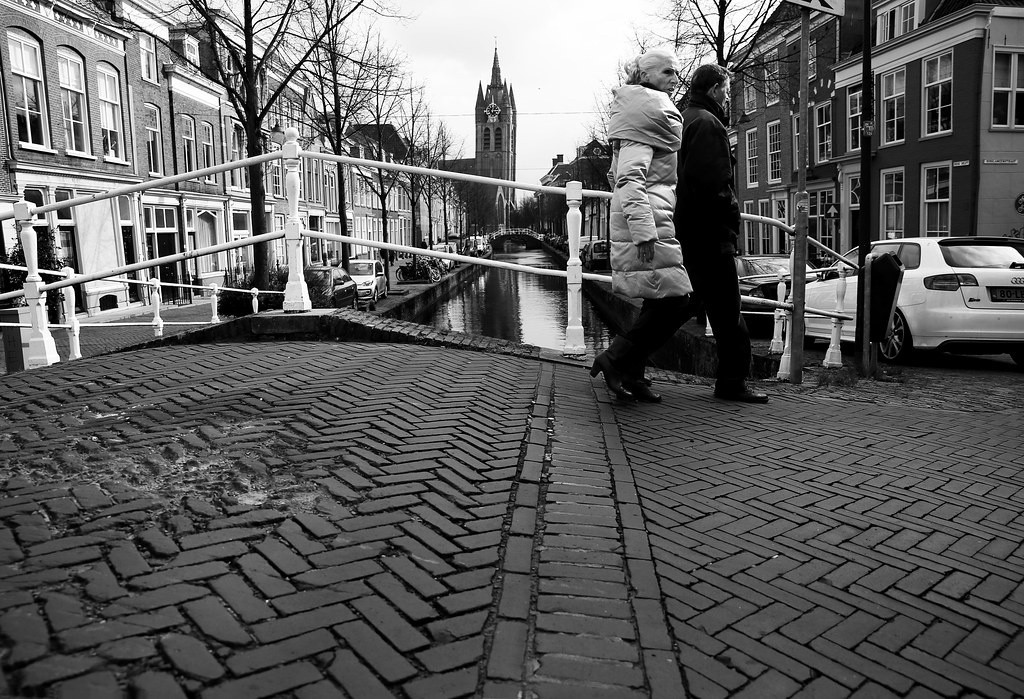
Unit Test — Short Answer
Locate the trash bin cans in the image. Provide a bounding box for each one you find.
[854,251,906,342]
[0,310,24,375]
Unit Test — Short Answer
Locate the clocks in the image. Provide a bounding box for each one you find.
[483,103,501,118]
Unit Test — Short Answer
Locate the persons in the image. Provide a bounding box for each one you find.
[380,249,396,266]
[421,238,427,249]
[589,50,771,404]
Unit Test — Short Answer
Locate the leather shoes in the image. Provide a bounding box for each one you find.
[643,377,652,386]
[713,378,769,403]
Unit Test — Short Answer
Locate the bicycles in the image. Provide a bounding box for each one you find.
[396,256,448,282]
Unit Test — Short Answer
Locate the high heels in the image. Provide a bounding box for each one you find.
[590,335,637,403]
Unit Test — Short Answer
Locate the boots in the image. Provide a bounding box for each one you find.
[617,362,662,402]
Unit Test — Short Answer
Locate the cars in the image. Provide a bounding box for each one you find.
[581,240,611,271]
[804,235,1024,366]
[735,253,822,338]
[426,236,489,272]
[338,259,388,303]
[305,267,359,311]
